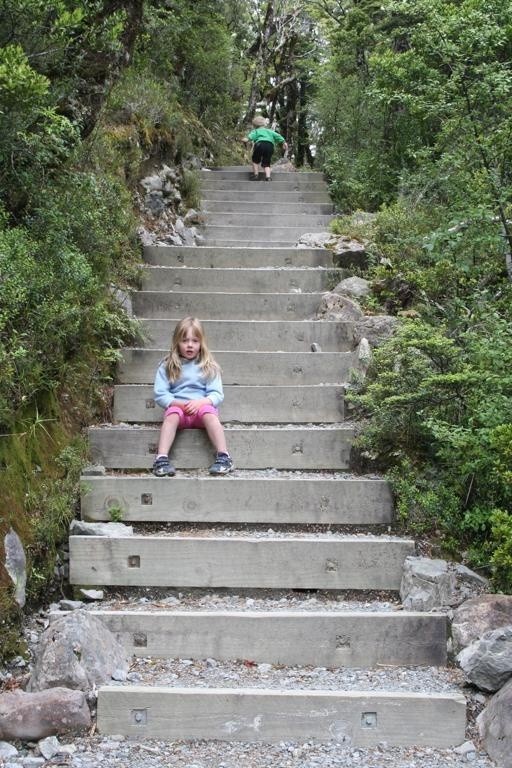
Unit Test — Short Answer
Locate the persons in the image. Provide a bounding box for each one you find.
[239,116,289,182]
[148,315,235,476]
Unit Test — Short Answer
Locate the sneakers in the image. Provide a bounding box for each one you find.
[210,451,234,475]
[152,456,175,476]
[250,175,272,181]
